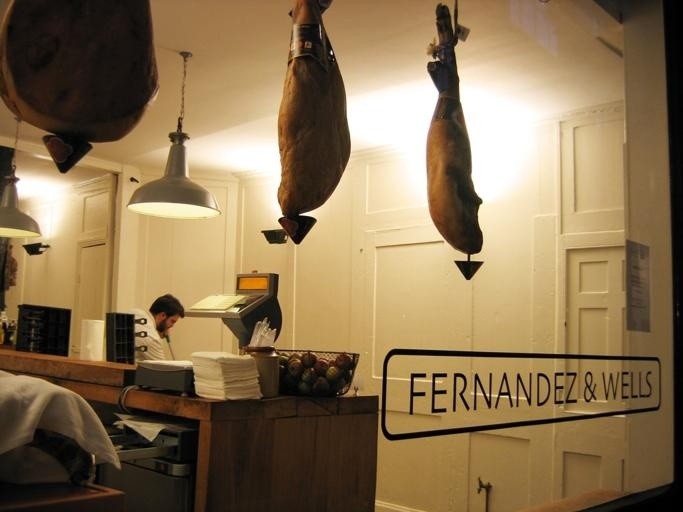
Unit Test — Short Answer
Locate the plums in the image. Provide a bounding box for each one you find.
[280,352,352,394]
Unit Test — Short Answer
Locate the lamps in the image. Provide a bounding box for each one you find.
[0,119,40,239]
[123,51,223,220]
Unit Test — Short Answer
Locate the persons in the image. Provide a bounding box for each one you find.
[127,293,184,361]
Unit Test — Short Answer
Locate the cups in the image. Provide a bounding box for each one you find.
[79,318,106,363]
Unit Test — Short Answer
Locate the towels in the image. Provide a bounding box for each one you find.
[1,370,124,471]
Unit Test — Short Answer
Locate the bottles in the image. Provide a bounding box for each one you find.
[243,345,281,400]
